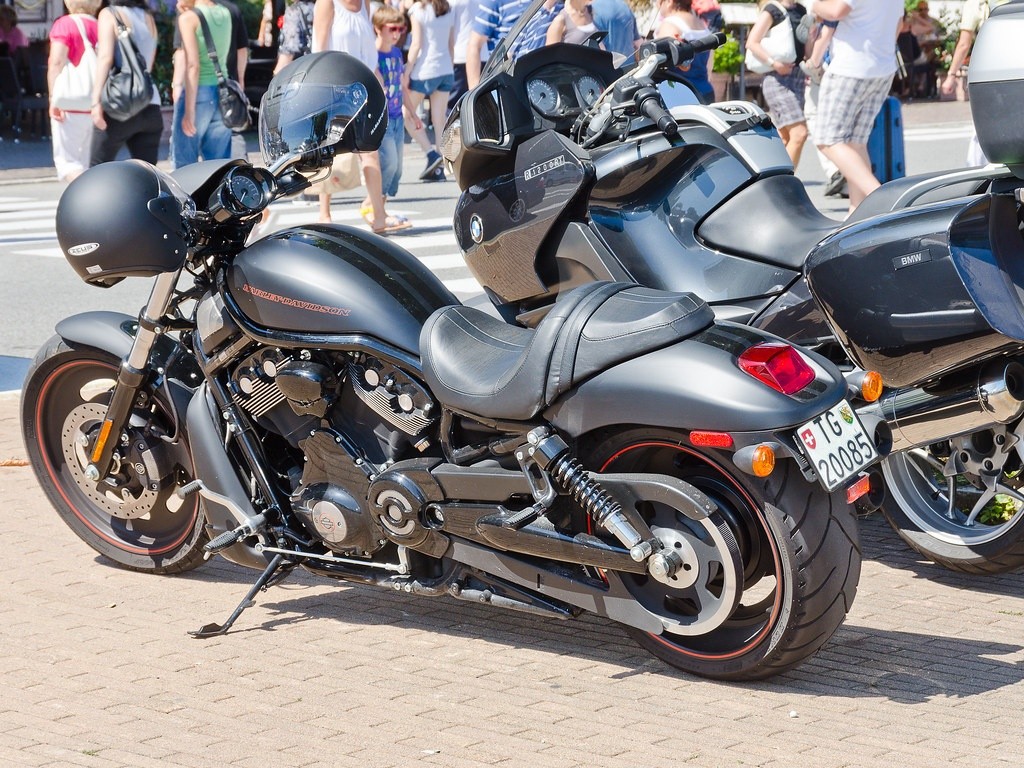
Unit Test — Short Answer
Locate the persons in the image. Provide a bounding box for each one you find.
[745,0,809,170]
[942,0,1007,167]
[799,0,906,216]
[47,0,102,183]
[171,0,274,237]
[89,0,164,168]
[272,0,715,233]
[0,5,28,51]
[897,1,941,96]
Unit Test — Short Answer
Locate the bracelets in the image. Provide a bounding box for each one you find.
[947,72,955,76]
[768,59,774,67]
[91,100,100,108]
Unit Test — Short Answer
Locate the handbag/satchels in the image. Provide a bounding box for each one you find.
[745,2,796,73]
[218,80,251,133]
[51,15,98,111]
[102,5,154,122]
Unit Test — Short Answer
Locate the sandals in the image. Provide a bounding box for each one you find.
[799,58,825,85]
[359,203,375,226]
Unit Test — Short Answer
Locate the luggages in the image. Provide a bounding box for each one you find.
[867,96,905,185]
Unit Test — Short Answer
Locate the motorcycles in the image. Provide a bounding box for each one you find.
[21,149,892,680]
[418,0,1024,576]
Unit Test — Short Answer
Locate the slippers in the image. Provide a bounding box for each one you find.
[372,215,412,233]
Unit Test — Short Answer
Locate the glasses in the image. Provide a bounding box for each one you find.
[382,25,408,35]
[918,7,928,12]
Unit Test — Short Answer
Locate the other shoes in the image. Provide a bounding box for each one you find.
[825,171,846,197]
[422,167,447,181]
[418,151,443,179]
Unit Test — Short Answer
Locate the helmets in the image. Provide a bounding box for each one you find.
[56,160,186,288]
[258,51,389,173]
